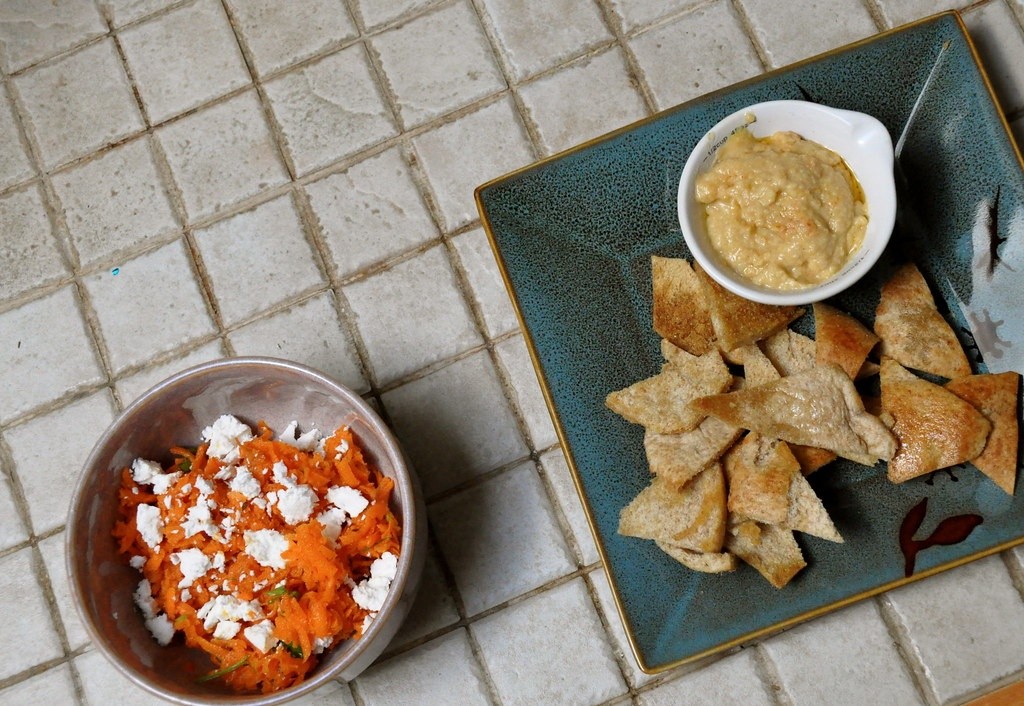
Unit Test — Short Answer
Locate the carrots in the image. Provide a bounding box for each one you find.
[112,427,400,696]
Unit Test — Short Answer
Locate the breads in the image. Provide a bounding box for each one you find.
[607,255,1020,590]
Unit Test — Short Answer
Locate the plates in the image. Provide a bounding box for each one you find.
[473,8,1024,677]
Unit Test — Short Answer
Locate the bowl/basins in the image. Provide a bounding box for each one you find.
[59,356,428,704]
[676,98,898,306]
